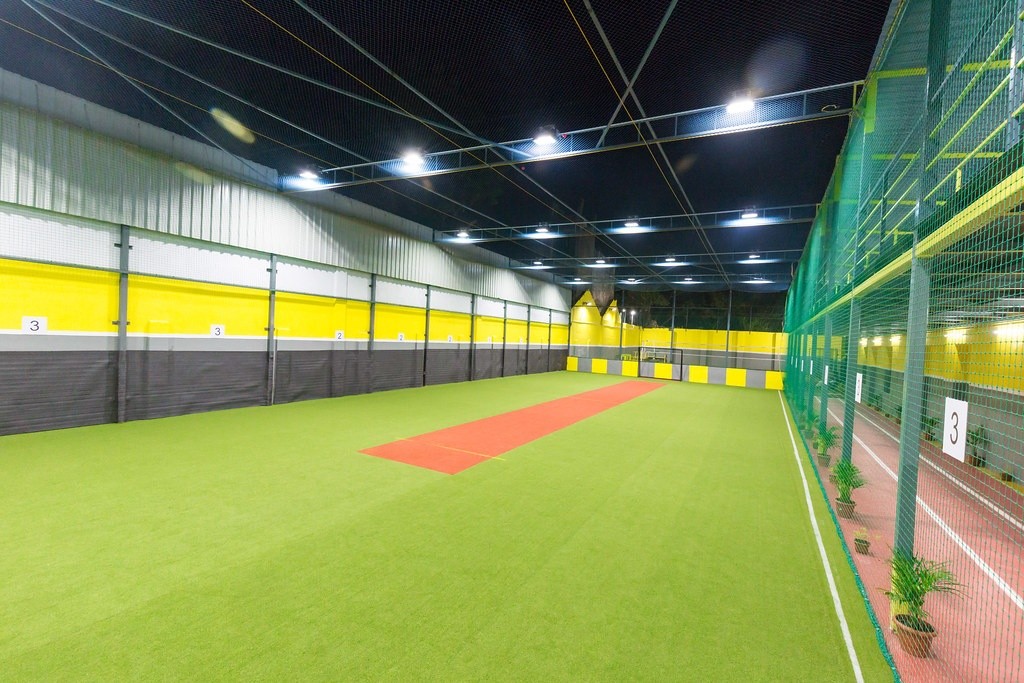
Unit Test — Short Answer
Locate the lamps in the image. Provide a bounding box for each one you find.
[573,274,581,280]
[741,205,759,219]
[299,163,323,179]
[624,215,639,228]
[665,251,676,261]
[628,272,635,280]
[532,124,556,146]
[754,273,762,280]
[684,274,692,280]
[457,226,469,238]
[596,255,606,264]
[534,257,543,265]
[535,222,549,232]
[749,249,760,259]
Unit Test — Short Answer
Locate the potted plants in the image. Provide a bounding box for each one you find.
[781,373,940,518]
[965,425,990,466]
[877,543,969,658]
[852,526,872,555]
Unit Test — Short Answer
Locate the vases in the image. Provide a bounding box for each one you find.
[1001,473,1012,481]
